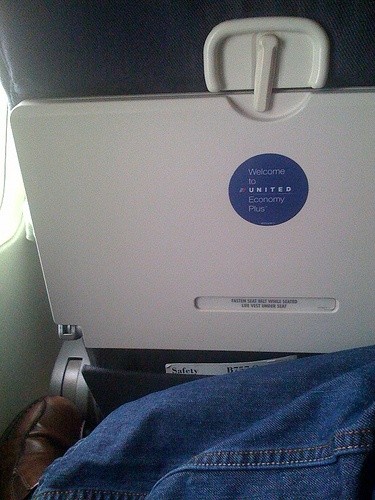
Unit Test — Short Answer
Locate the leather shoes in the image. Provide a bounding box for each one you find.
[0,396,82,500]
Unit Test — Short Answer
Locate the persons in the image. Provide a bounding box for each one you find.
[0,340,375,498]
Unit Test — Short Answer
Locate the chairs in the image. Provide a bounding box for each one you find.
[1,0,374,440]
